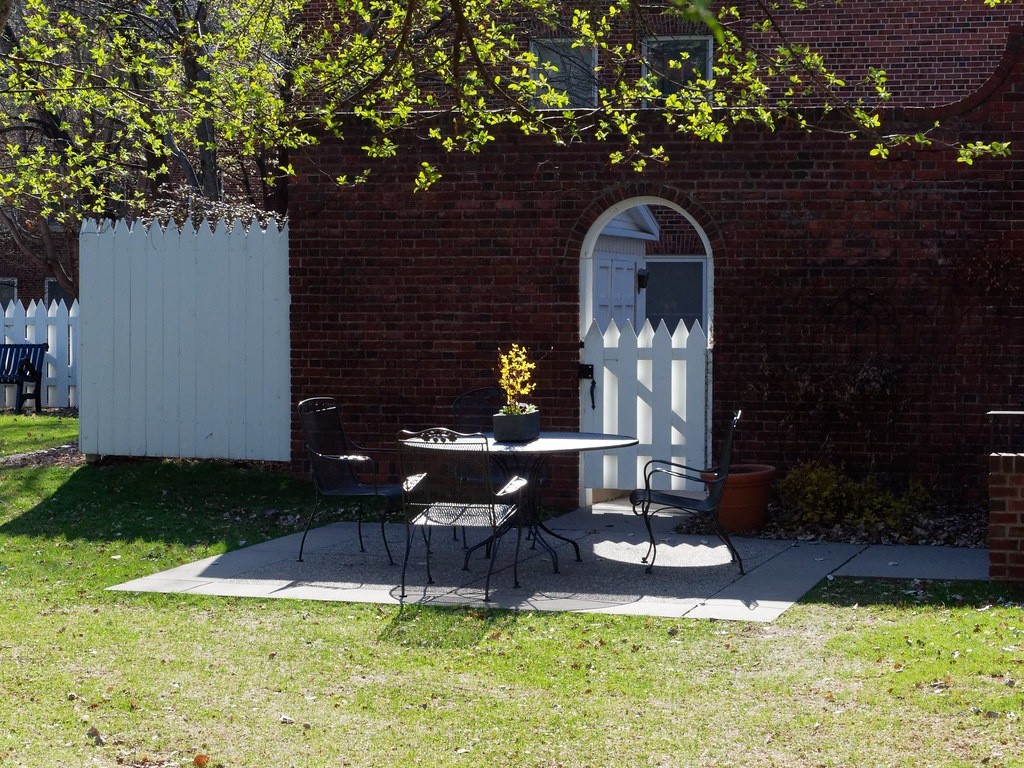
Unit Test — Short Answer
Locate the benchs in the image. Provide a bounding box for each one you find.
[0,342,50,413]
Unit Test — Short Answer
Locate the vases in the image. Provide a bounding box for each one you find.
[701,462,780,534]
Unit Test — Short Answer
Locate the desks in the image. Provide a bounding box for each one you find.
[397,432,641,590]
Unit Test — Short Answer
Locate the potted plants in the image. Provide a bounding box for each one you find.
[481,344,556,445]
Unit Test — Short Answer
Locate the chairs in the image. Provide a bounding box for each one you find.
[452,388,542,550]
[296,397,406,563]
[630,406,748,576]
[398,427,523,598]
